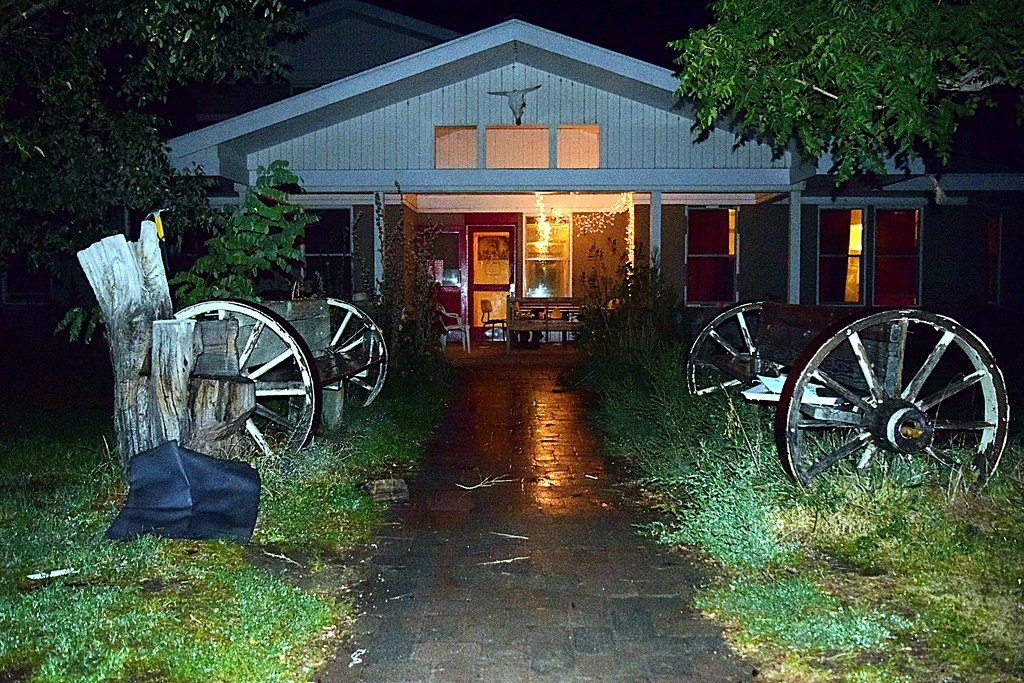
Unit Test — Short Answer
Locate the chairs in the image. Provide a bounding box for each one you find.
[481,300,506,344]
[436,303,470,354]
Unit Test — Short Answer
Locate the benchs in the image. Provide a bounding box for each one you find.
[508,297,588,349]
